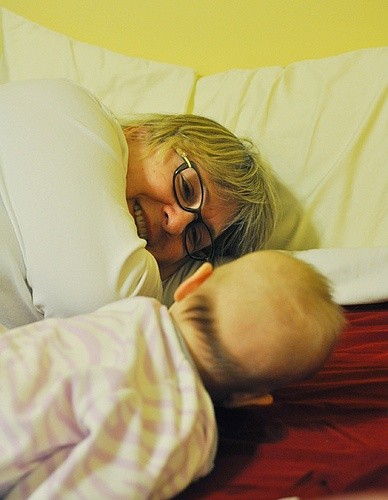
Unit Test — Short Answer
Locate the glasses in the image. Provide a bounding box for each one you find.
[171,143,214,261]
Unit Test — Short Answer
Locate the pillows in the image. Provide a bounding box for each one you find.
[0,4,388,251]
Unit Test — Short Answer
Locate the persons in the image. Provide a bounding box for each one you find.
[0,250,347,500]
[0,81,274,334]
